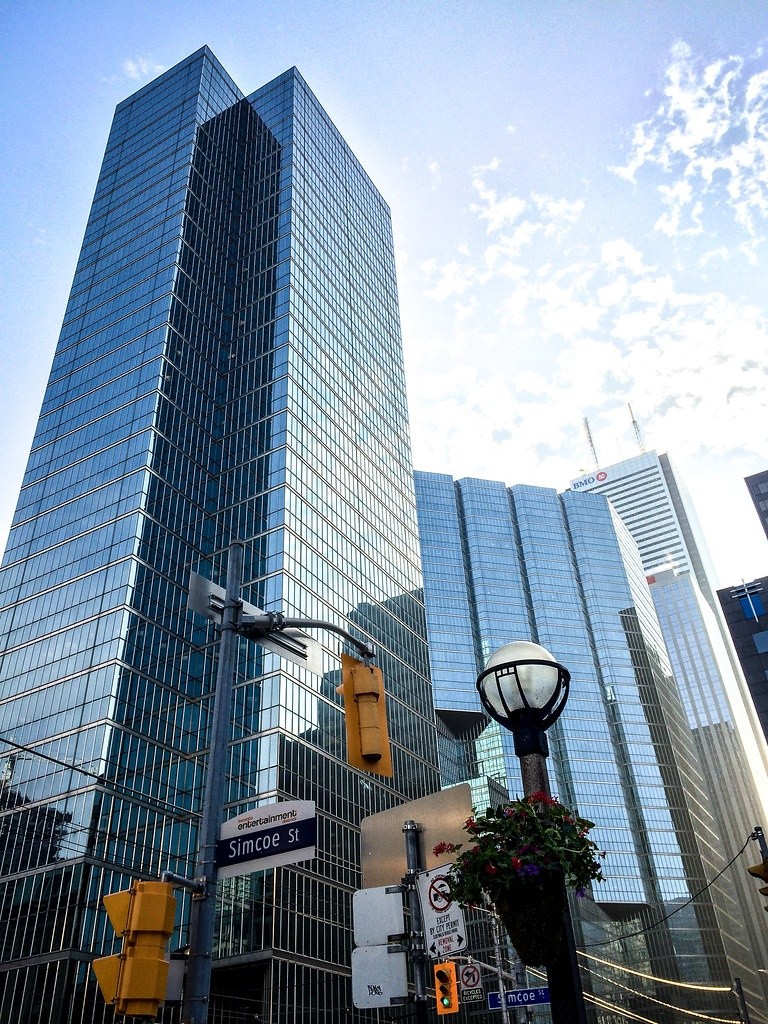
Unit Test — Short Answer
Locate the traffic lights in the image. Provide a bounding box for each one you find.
[434,962,459,1015]
[336,652,392,776]
[747,860,768,911]
[92,879,177,1019]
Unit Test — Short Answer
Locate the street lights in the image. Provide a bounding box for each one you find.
[477,643,602,1024]
